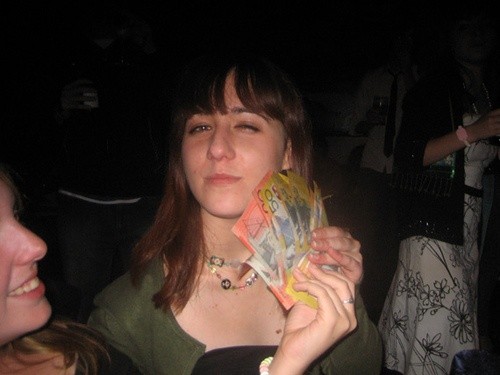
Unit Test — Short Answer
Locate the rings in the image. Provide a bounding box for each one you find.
[344,299,355,304]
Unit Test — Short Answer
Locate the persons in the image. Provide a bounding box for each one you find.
[1,172,142,375]
[87,49,384,375]
[342,17,418,184]
[378,0,500,374]
[50,0,164,312]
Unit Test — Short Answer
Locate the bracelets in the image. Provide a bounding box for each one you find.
[456,127,470,147]
[258,357,273,375]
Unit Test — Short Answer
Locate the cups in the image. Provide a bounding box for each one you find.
[372,95,388,115]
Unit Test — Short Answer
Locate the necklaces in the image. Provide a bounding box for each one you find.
[205,255,258,289]
[462,78,492,116]
